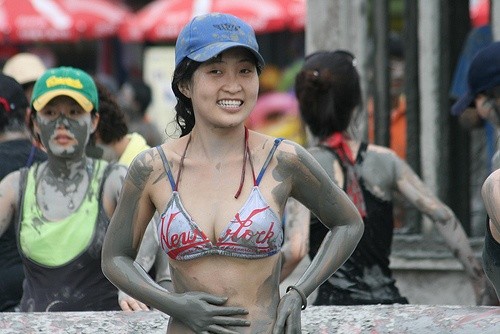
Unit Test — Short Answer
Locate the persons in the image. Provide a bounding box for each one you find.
[0,51,500,334]
[467,39,500,307]
[101,12,365,334]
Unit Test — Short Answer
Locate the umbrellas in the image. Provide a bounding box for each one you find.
[117,0,308,43]
[0,0,131,40]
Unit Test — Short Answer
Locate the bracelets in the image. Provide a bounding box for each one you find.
[285,285,307,311]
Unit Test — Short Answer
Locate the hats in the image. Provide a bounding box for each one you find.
[175,13,265,67]
[2,53,47,85]
[30,66,99,113]
[0,74,30,111]
[451,41,500,115]
